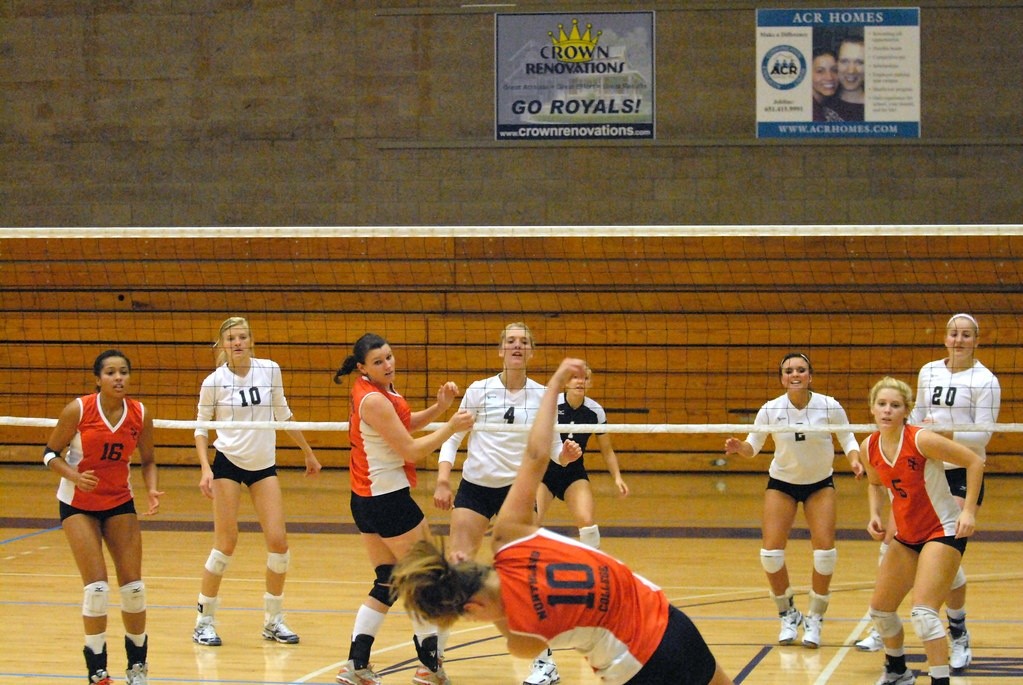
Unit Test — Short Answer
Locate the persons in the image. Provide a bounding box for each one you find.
[43,350,165,685]
[857,312,1002,674]
[724,352,864,649]
[859,377,984,685]
[536,360,629,549]
[334,333,474,685]
[813,36,865,122]
[392,357,734,685]
[192,317,322,646]
[435,323,582,685]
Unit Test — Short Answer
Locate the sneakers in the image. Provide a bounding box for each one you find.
[336,659,380,685]
[856,630,883,652]
[88,671,109,685]
[949,635,972,670]
[778,611,803,643]
[876,668,916,684]
[125,662,148,685]
[523,658,560,685]
[263,613,299,644]
[802,616,820,648]
[413,665,452,685]
[193,616,222,646]
